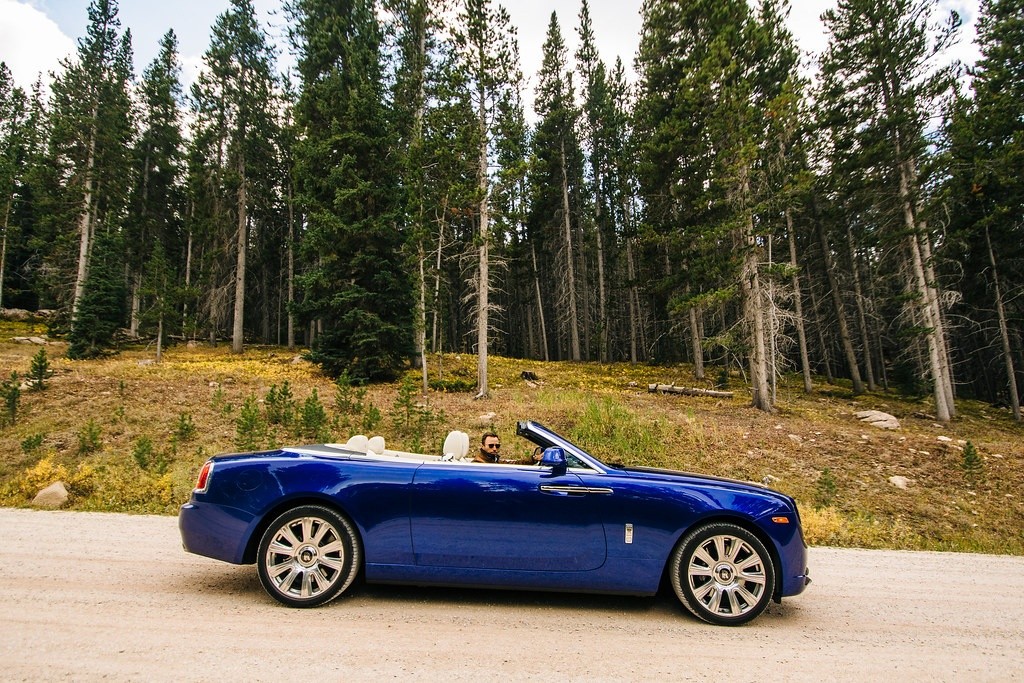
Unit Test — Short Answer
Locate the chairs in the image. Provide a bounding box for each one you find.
[369,435,384,455]
[345,435,369,456]
[462,433,469,456]
[443,431,464,462]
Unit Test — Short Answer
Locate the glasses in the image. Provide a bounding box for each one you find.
[485,444,500,448]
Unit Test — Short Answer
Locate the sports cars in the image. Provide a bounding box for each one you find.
[178,420,812,629]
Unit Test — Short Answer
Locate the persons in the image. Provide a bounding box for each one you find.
[472,432,544,465]
